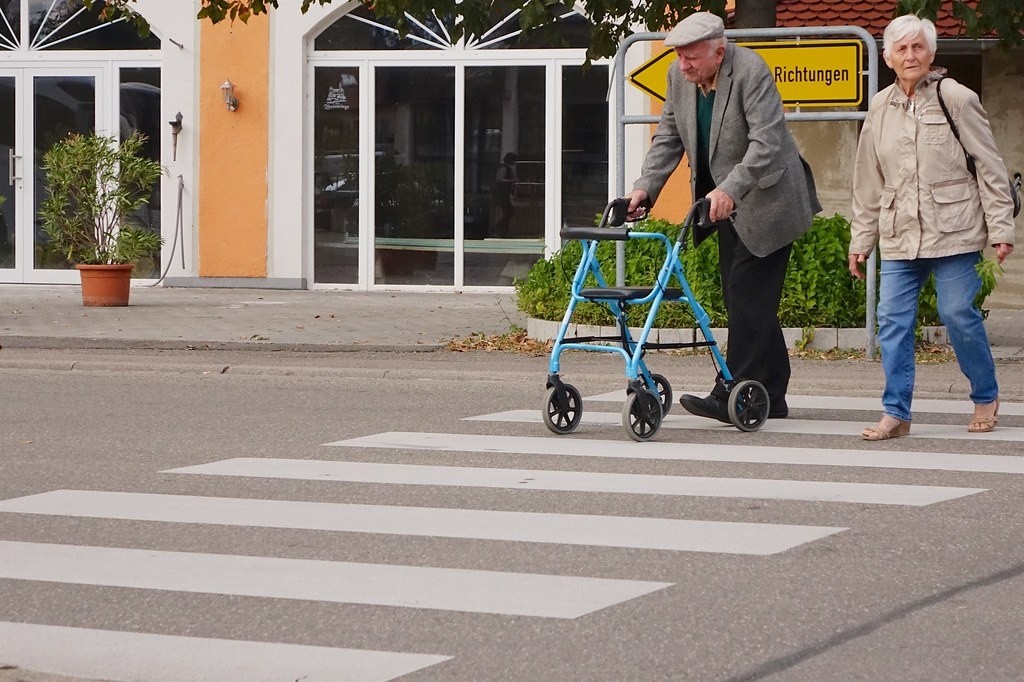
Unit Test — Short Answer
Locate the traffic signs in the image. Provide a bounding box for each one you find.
[624,37,864,108]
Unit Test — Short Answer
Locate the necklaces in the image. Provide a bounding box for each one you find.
[700,66,719,98]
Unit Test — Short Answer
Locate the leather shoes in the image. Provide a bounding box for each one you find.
[749,396,787,420]
[679,395,732,424]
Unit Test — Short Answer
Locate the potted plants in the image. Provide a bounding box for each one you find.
[35,129,171,307]
[379,159,446,275]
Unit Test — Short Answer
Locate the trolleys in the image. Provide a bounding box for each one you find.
[536,197,772,443]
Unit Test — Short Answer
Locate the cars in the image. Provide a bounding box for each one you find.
[312,152,466,240]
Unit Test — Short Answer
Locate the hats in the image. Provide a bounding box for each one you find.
[664,12,725,46]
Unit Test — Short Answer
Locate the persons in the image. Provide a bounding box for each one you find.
[622,11,823,424]
[848,14,1015,440]
[492,152,519,238]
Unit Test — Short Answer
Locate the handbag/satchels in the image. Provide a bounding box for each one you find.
[966,154,1022,217]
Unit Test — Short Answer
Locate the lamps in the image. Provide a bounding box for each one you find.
[219,77,239,112]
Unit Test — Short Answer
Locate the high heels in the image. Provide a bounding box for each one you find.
[861,415,912,440]
[968,396,1000,432]
